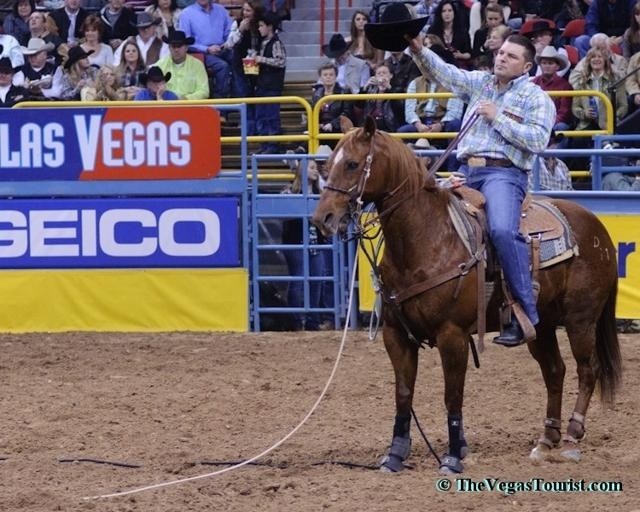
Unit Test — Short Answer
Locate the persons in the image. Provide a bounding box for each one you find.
[309,142,338,333]
[280,156,326,334]
[0,1,639,193]
[402,30,560,349]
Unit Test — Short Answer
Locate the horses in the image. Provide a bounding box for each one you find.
[309,113,623,477]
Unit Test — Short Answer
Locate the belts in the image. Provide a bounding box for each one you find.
[465,157,513,167]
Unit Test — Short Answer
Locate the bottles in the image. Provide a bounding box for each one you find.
[588,96,599,120]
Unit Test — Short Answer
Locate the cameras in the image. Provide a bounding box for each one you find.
[375,78,386,85]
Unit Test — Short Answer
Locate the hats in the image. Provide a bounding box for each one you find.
[63,47,94,69]
[364,3,429,52]
[130,12,161,28]
[19,37,57,55]
[524,21,564,38]
[162,31,194,45]
[0,58,24,73]
[251,10,282,32]
[534,45,568,71]
[139,66,171,86]
[322,34,353,57]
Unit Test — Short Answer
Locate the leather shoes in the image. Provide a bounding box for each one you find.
[493,319,540,346]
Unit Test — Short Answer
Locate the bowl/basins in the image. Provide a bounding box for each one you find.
[242,58,261,75]
